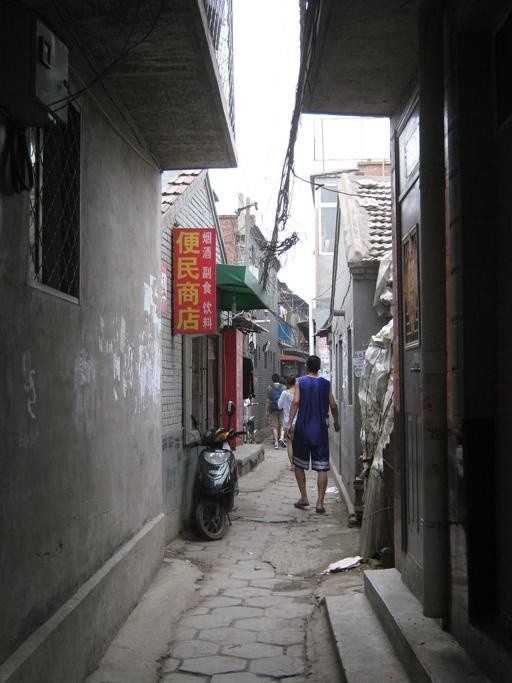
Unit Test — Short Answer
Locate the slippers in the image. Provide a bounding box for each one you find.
[295,501,310,507]
[316,507,326,513]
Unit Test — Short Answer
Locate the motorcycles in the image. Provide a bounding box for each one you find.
[187,401,246,540]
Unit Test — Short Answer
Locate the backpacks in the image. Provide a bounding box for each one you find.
[270,384,284,412]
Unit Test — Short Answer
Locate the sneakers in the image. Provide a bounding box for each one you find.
[274,444,279,448]
[279,439,287,447]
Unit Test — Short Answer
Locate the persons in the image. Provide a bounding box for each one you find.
[278,373,299,471]
[287,354,341,513]
[265,372,302,449]
